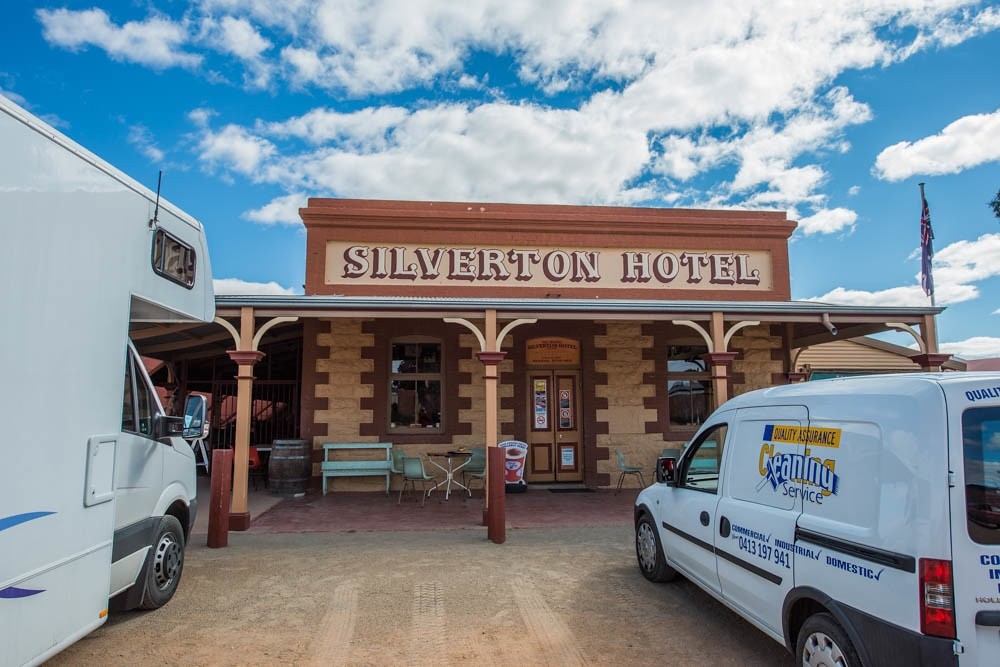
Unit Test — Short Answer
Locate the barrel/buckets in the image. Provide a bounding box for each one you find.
[267,439,313,498]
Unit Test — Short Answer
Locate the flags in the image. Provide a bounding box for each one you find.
[921,200,935,297]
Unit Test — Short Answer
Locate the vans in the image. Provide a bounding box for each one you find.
[634,371,1000,667]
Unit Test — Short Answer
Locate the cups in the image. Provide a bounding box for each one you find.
[504,446,527,482]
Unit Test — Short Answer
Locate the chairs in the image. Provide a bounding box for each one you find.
[391,450,442,508]
[615,447,646,497]
[652,449,680,484]
[230,445,268,493]
[463,447,487,500]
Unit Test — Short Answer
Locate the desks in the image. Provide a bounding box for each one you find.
[427,452,472,501]
[257,446,273,451]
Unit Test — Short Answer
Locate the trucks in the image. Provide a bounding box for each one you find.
[0,92,217,667]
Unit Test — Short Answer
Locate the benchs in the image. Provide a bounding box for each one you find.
[320,442,393,496]
[684,441,718,468]
